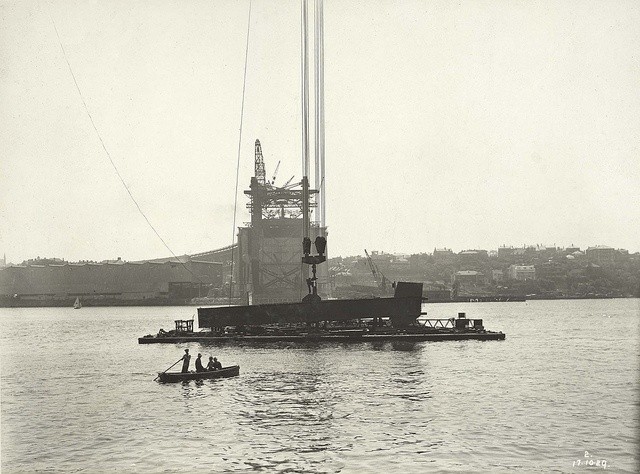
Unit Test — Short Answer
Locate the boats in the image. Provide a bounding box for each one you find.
[158,365,240,383]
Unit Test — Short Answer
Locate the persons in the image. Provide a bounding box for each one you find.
[195,353,206,371]
[181,349,191,372]
[205,357,215,371]
[214,358,222,371]
